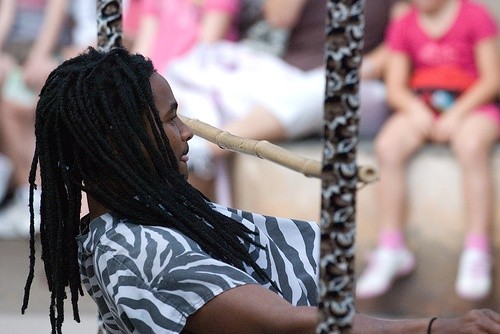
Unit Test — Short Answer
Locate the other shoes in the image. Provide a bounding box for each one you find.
[186,136,216,183]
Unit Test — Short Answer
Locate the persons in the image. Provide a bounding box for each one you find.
[0,1,411,238]
[355,1,499,301]
[21,46,500,334]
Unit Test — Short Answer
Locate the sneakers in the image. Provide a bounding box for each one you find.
[354,248,494,302]
[0,151,41,242]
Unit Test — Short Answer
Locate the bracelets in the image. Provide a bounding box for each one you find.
[427,317,438,334]
